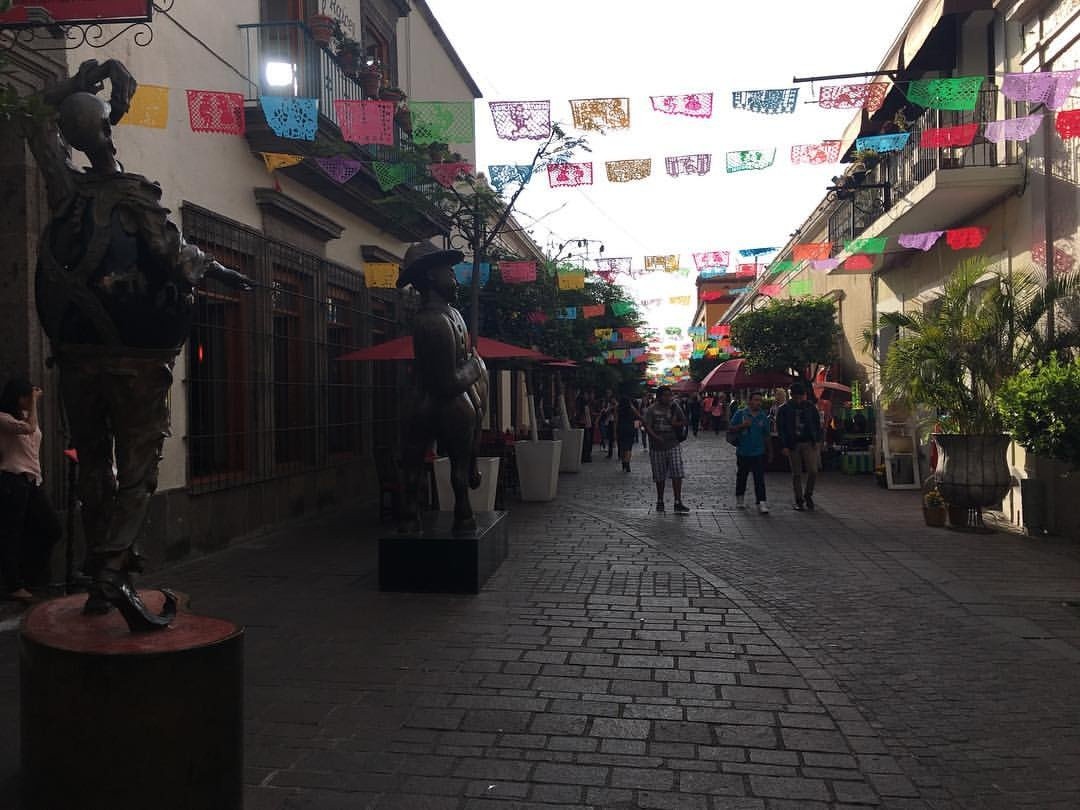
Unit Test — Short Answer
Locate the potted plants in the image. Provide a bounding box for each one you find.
[379,87,408,108]
[856,254,1080,528]
[850,150,879,180]
[372,125,647,515]
[335,39,360,65]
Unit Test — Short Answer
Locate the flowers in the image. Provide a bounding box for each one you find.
[359,63,386,72]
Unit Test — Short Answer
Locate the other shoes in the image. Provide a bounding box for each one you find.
[793,504,803,510]
[656,503,664,512]
[674,504,690,512]
[737,501,746,508]
[757,501,768,512]
[804,494,816,510]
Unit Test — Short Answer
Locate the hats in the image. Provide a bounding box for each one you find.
[791,383,806,394]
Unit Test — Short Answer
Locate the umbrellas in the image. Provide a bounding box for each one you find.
[335,331,581,432]
[653,359,802,393]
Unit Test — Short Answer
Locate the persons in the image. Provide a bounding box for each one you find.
[25,53,210,633]
[569,383,866,514]
[0,380,57,602]
[396,240,492,530]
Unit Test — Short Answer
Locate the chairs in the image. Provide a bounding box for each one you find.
[373,444,399,520]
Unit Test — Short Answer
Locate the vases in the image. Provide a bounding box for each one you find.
[360,71,380,96]
[311,13,337,46]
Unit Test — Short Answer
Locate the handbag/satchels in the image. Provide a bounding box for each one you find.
[725,430,741,446]
[671,403,688,442]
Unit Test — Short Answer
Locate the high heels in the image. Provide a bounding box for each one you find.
[599,441,606,449]
[622,462,632,472]
